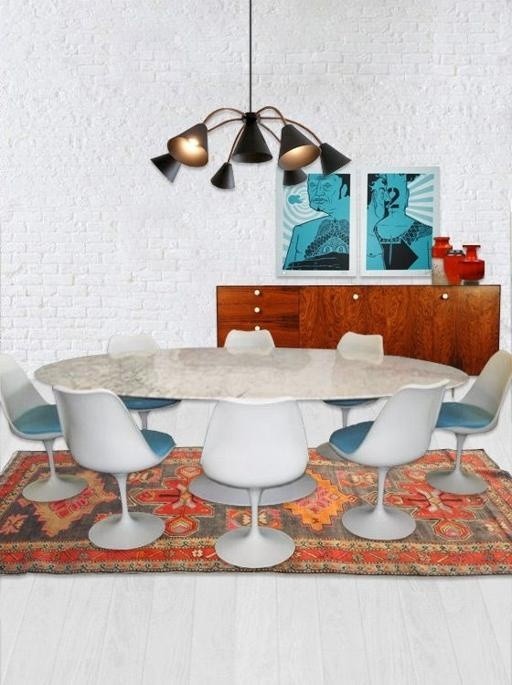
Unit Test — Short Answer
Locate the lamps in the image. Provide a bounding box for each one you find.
[148,0,351,189]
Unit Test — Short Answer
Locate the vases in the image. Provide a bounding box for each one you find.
[445,249,465,284]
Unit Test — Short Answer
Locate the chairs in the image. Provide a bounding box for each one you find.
[328,377,452,541]
[305,331,384,461]
[0,352,89,502]
[425,348,512,495]
[223,330,275,347]
[185,398,317,568]
[51,385,176,551]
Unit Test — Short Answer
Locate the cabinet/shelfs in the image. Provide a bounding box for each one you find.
[215,284,501,377]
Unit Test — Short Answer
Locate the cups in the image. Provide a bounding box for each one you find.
[443,255,464,283]
[448,250,463,256]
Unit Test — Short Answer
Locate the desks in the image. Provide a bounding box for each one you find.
[31,346,469,506]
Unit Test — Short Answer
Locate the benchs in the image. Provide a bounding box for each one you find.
[107,335,182,430]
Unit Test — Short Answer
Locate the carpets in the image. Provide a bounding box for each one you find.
[1,447,512,577]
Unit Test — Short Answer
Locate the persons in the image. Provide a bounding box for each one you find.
[281,173,349,271]
[365,173,433,270]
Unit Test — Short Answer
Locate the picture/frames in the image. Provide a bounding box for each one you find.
[359,166,446,278]
[275,164,358,278]
